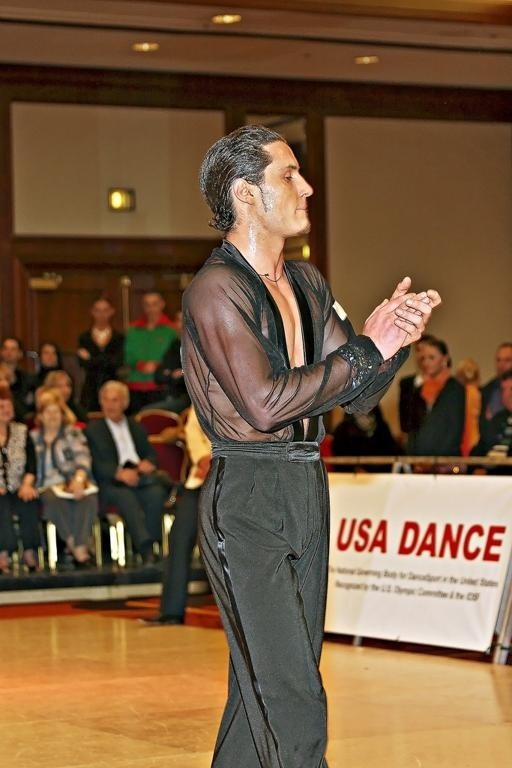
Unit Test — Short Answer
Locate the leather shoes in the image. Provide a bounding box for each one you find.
[68,552,159,572]
[137,614,184,624]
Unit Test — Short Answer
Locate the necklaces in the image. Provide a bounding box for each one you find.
[257,273,285,281]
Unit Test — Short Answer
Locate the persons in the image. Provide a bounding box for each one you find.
[1,287,213,626]
[329,335,512,475]
[182,124,442,768]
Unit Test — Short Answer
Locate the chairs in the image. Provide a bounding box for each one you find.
[4,407,202,578]
